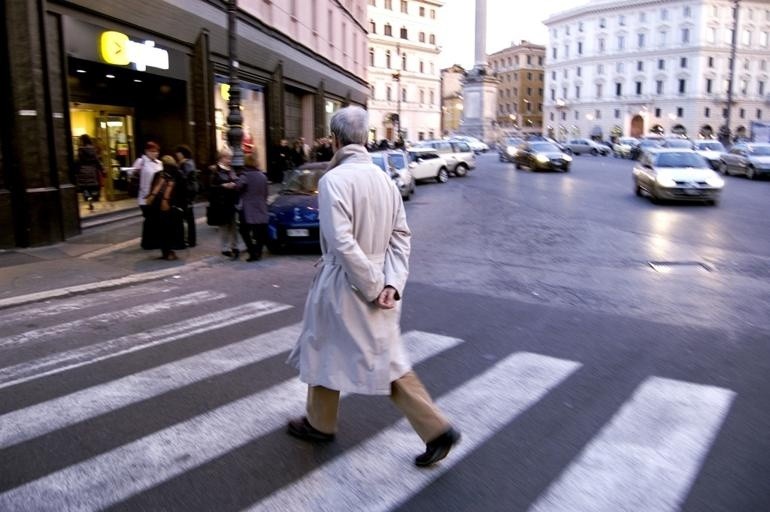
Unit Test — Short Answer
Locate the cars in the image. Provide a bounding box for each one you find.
[629,148,726,204]
[405,146,451,184]
[385,148,416,199]
[515,140,572,173]
[263,160,332,253]
[531,133,728,172]
[496,135,526,162]
[366,151,402,196]
[718,143,770,179]
[450,132,490,152]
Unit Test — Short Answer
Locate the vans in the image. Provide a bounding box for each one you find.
[416,140,477,177]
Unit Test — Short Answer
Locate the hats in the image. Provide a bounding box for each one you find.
[143,141,259,170]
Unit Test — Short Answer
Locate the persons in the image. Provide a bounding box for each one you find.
[288,104,462,467]
[74,133,408,262]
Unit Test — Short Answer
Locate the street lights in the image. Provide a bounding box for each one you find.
[393,66,403,138]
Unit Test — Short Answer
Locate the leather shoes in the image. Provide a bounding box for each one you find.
[286,417,338,445]
[158,240,262,261]
[414,427,462,467]
